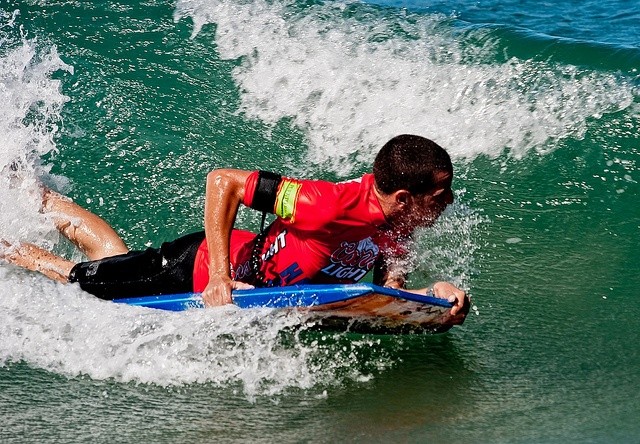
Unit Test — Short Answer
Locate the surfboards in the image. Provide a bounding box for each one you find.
[114,283,455,334]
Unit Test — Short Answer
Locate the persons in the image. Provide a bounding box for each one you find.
[0,134,470,326]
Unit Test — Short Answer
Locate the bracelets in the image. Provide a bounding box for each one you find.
[427,282,434,298]
